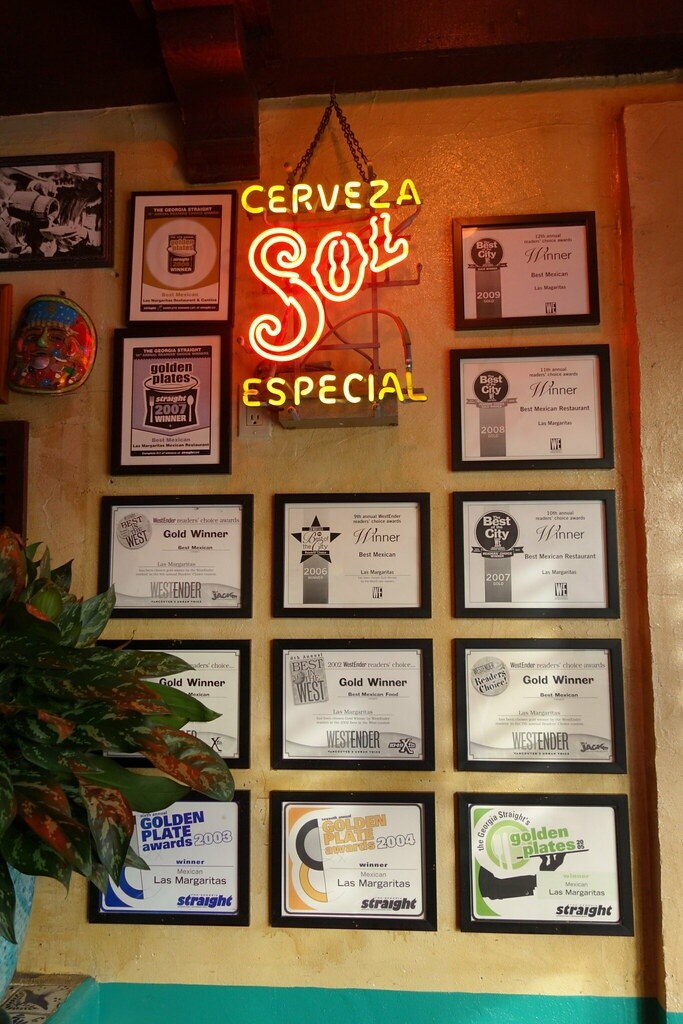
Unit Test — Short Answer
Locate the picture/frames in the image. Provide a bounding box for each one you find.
[0,149,116,272]
[271,491,432,620]
[269,637,435,772]
[96,493,254,619]
[453,790,635,938]
[268,789,438,932]
[449,343,615,473]
[452,210,601,331]
[123,189,238,326]
[453,638,629,775]
[85,790,252,927]
[94,637,251,770]
[109,325,232,477]
[452,489,621,620]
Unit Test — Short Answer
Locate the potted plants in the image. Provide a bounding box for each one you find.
[0,529,235,1003]
